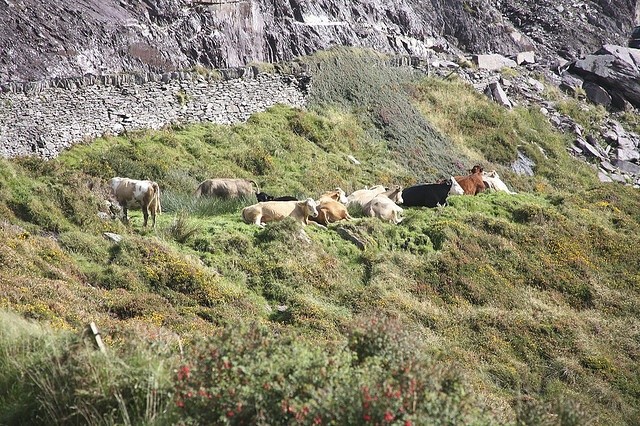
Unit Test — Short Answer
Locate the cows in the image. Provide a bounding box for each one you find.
[363,194,405,225]
[402,177,465,210]
[346,181,409,219]
[311,188,354,224]
[250,191,297,203]
[191,177,260,201]
[457,165,486,195]
[241,197,321,227]
[107,177,161,228]
[485,170,516,195]
